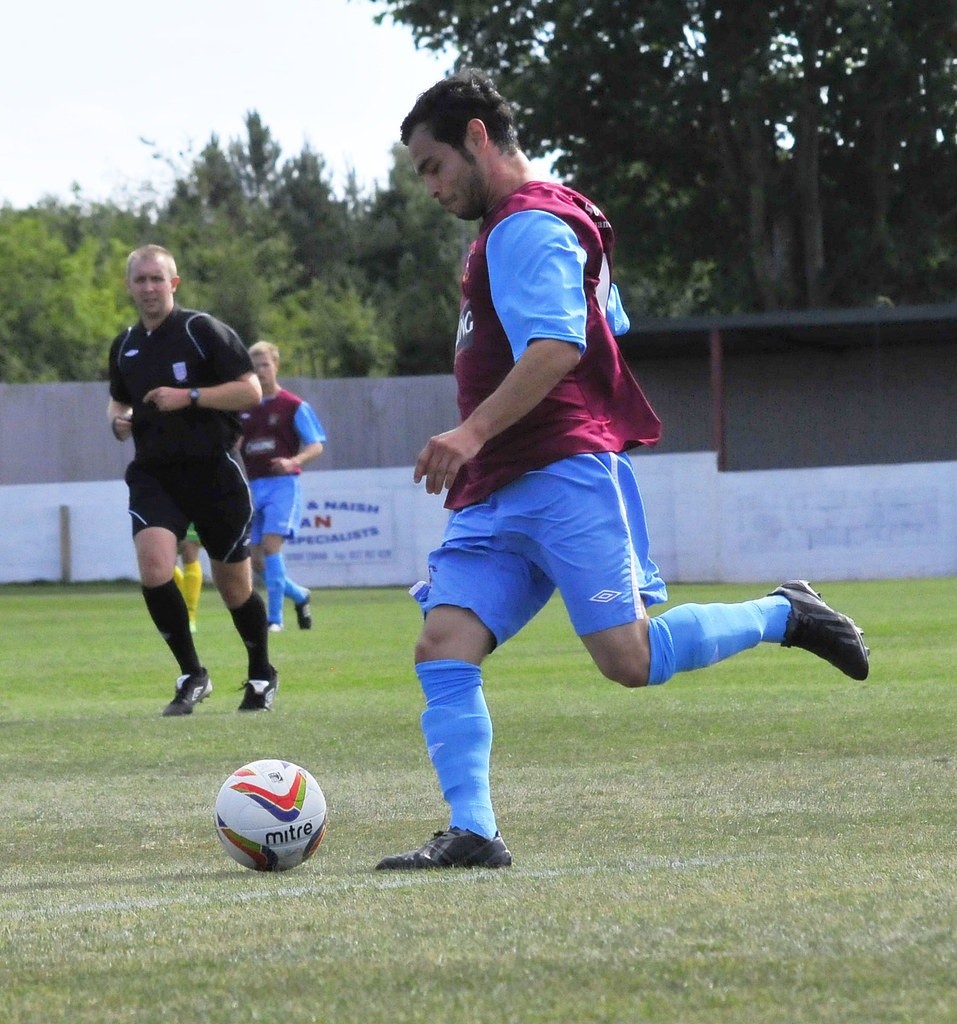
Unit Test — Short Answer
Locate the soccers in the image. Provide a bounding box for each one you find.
[213,758,329,874]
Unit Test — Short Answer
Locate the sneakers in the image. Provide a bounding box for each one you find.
[267,621,284,633]
[375,824,512,873]
[163,666,212,715]
[293,587,313,629]
[765,578,870,682]
[238,664,279,714]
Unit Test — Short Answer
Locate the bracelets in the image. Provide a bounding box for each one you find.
[112,416,122,441]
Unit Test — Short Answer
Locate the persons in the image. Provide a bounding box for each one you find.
[171,522,205,635]
[235,341,327,630]
[108,244,279,716]
[375,69,870,867]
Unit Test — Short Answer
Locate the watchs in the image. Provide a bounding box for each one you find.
[189,387,200,408]
